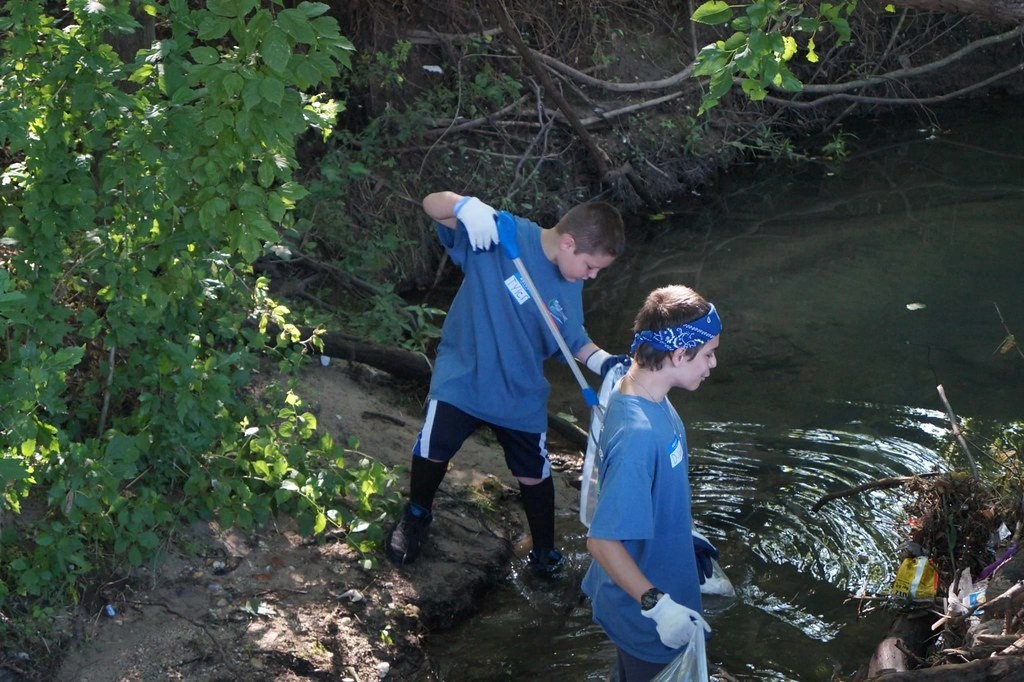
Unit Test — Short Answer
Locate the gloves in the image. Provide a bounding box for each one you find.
[641,593,711,649]
[692,530,718,585]
[586,349,632,380]
[454,196,500,251]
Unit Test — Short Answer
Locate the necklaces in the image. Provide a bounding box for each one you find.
[628,369,683,446]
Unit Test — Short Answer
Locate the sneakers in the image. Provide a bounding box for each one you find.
[384,502,433,561]
[522,550,584,586]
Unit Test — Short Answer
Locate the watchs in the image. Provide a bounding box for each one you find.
[640,588,665,611]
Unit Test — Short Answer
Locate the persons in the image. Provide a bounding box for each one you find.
[580,284,723,681]
[384,191,634,580]
